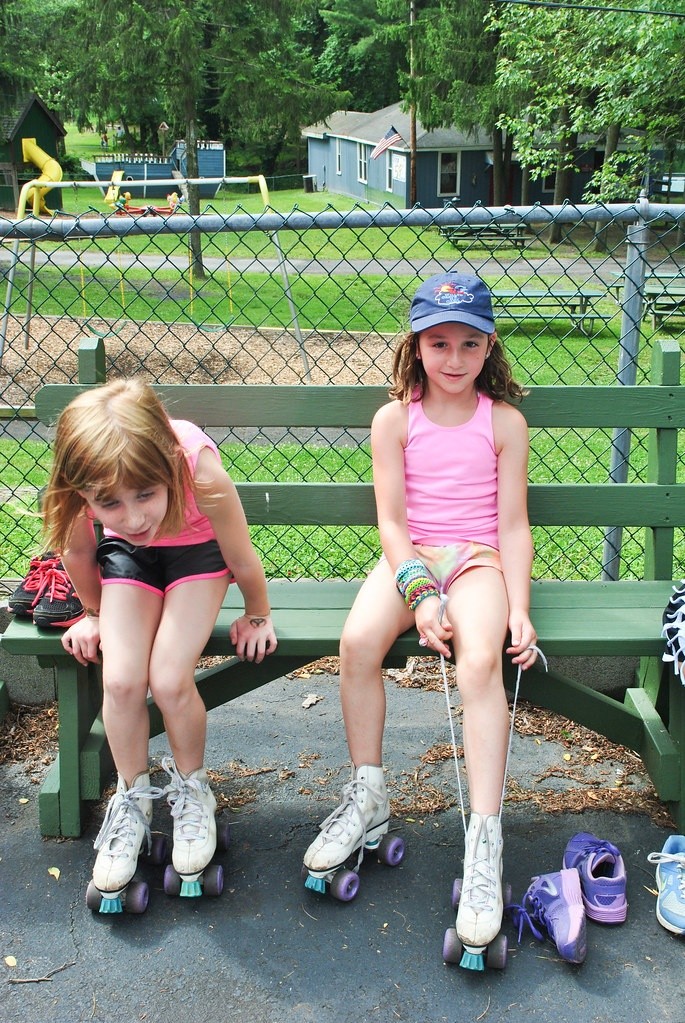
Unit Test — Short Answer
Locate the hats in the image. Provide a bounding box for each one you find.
[410,271,495,335]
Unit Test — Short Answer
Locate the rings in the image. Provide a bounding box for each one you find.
[419,637,429,646]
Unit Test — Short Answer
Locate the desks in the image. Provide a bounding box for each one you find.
[440,223,527,247]
[489,289,607,335]
[644,284,685,330]
[610,271,685,282]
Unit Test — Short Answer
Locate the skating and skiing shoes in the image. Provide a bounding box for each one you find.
[300,762,405,901]
[438,591,547,968]
[86,769,166,913]
[162,754,230,897]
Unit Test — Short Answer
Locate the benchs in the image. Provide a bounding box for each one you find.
[440,231,531,241]
[2,339,685,838]
[649,310,685,317]
[492,312,612,332]
[643,302,685,307]
[493,302,591,314]
[606,282,623,305]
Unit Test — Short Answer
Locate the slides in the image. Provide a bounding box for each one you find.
[20,137,64,215]
[105,170,124,202]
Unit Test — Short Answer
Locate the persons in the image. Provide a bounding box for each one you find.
[299,273,538,972]
[39,378,278,914]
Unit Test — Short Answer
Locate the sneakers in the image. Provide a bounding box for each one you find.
[562,831,627,923]
[504,868,587,964]
[647,834,685,935]
[7,545,86,629]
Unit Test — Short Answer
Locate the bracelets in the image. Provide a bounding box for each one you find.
[394,559,439,610]
